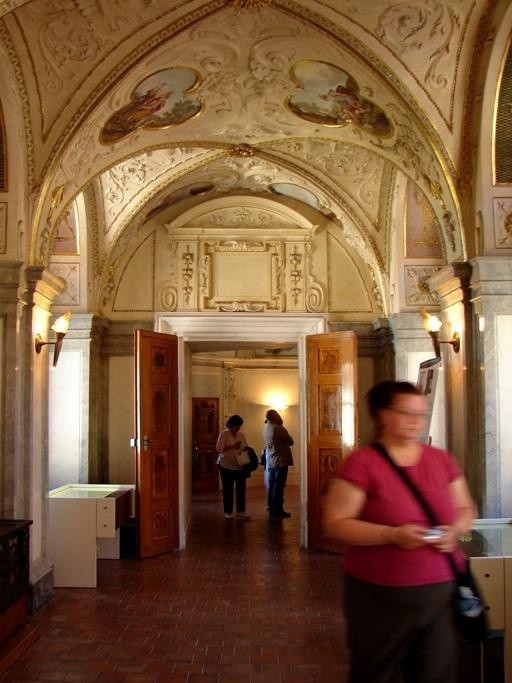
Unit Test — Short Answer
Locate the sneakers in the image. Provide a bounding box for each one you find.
[225,514,232,518]
[234,512,251,521]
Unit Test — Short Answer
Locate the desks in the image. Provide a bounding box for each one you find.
[44,481,141,590]
[0,516,37,638]
[464,513,511,682]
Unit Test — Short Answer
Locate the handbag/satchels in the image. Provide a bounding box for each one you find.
[451,569,494,651]
[235,451,250,466]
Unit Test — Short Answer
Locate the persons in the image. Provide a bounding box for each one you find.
[317,377,485,681]
[263,408,294,518]
[214,412,253,522]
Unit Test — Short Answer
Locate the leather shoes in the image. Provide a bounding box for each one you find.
[268,509,291,518]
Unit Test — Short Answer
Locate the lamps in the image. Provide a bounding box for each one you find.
[34,309,74,367]
[418,308,461,362]
